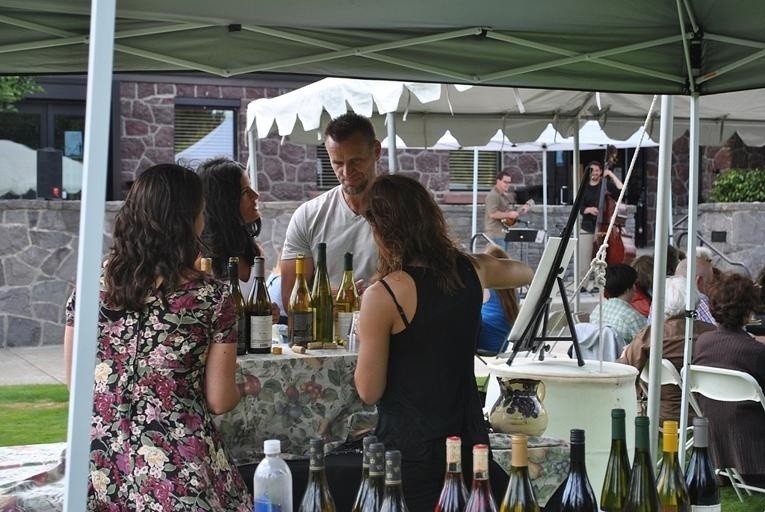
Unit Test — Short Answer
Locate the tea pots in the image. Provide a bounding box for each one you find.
[488,375,549,437]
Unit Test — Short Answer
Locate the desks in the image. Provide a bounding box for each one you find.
[483,431,578,512]
[209,342,380,471]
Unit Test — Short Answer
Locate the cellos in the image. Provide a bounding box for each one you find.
[593,144,637,266]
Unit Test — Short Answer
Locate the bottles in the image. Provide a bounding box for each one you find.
[252,439,293,511]
[347,434,407,511]
[630,415,660,511]
[500,436,540,510]
[297,437,338,512]
[684,414,721,510]
[193,242,361,353]
[597,408,630,509]
[655,420,690,509]
[545,430,598,510]
[467,443,499,511]
[433,434,466,512]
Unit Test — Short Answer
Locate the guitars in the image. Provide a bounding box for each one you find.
[502,199,535,227]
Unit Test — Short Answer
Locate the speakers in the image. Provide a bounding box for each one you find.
[37,146,61,200]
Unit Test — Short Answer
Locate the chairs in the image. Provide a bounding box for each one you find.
[680,364,765,505]
[569,308,591,322]
[638,357,753,498]
[574,324,622,363]
